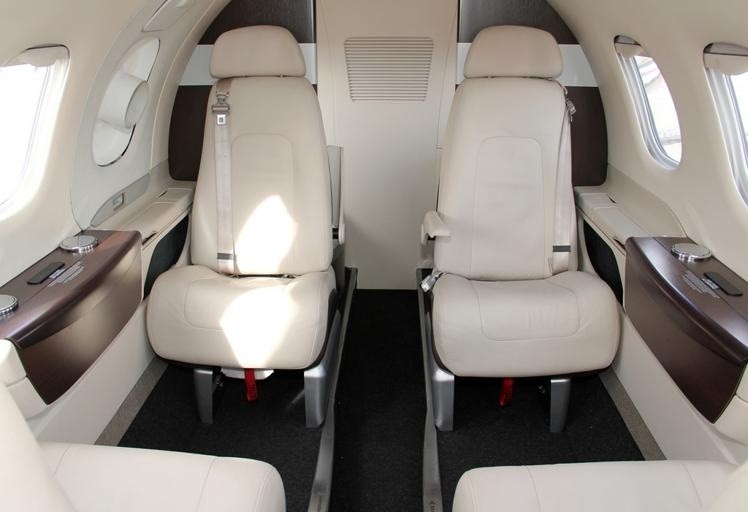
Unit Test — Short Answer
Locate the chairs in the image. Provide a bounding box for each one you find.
[0,371,289,512]
[147,22,347,429]
[413,24,622,436]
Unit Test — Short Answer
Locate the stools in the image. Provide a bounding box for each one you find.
[442,439,748,512]
